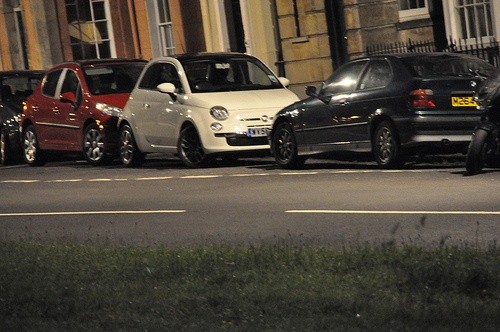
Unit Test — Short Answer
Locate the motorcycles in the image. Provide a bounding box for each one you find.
[465,62,500,175]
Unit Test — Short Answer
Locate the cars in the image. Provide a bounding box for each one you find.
[117,51,301,169]
[19,57,155,167]
[0,69,48,168]
[268,53,497,170]
